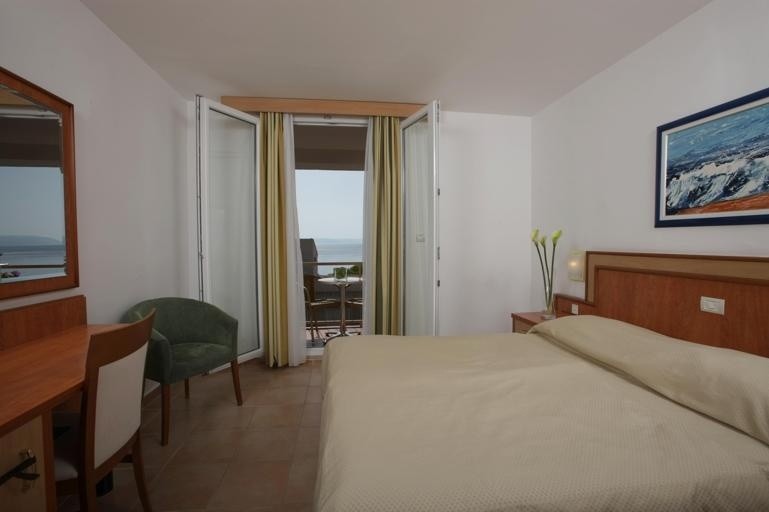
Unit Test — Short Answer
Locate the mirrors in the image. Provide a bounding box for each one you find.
[0,66,80,297]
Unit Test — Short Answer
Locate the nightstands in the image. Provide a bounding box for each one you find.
[510,313,546,335]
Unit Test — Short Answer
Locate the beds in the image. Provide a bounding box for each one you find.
[315,251,768,512]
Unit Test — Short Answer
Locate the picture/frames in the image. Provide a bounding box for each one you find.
[655,87,769,227]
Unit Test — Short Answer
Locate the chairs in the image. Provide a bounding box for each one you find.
[122,296,242,444]
[303,272,344,344]
[58,304,158,512]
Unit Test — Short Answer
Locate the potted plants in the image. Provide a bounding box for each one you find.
[532,229,561,319]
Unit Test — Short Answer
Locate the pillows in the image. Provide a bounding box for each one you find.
[542,316,769,445]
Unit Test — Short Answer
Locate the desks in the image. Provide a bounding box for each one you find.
[0,294,131,437]
[318,277,363,346]
